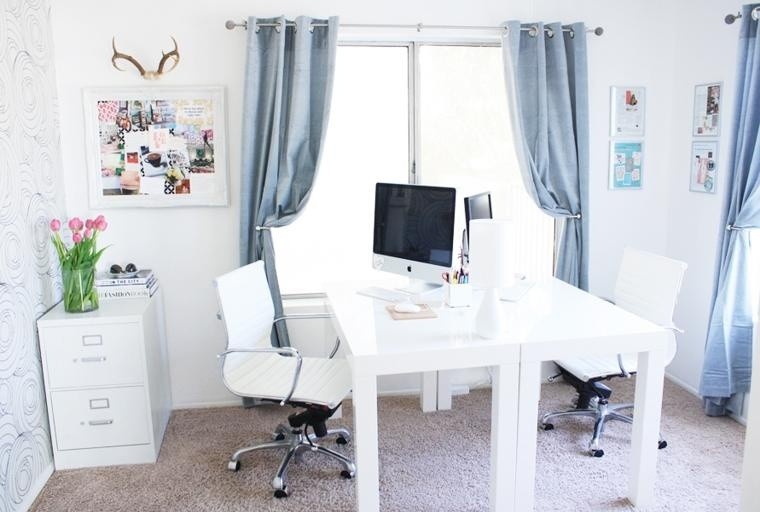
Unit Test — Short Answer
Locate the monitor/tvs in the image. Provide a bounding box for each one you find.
[464,190,493,261]
[372,183,456,293]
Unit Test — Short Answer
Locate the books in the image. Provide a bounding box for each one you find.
[93,268,161,301]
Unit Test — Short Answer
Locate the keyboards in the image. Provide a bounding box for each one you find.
[358,287,408,302]
[499,280,535,302]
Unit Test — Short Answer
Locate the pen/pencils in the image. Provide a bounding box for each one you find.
[446,268,468,284]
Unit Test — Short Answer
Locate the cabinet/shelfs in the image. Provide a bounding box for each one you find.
[35,292,171,472]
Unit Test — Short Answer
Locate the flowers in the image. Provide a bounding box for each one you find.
[51,216,111,312]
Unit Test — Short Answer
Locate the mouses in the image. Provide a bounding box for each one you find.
[395,302,420,313]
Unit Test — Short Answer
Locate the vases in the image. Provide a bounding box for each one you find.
[62,269,100,315]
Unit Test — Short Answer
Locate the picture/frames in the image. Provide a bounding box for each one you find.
[87,90,229,209]
[609,86,647,189]
[687,83,723,197]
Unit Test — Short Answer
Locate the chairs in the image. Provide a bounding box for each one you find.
[538,250,689,462]
[212,260,353,502]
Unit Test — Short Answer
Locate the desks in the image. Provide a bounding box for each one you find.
[321,254,668,512]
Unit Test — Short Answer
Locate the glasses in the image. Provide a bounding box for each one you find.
[110,264,136,274]
